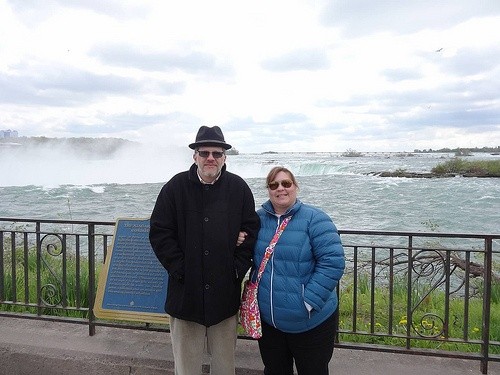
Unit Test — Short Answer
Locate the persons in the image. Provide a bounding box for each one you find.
[236,166,345,375]
[149,126,261,375]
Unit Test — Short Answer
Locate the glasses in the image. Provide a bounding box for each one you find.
[267,179,293,190]
[198,150,224,158]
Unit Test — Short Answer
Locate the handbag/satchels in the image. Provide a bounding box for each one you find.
[240,280,262,338]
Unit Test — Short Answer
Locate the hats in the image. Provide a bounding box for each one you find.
[189,126,231,150]
